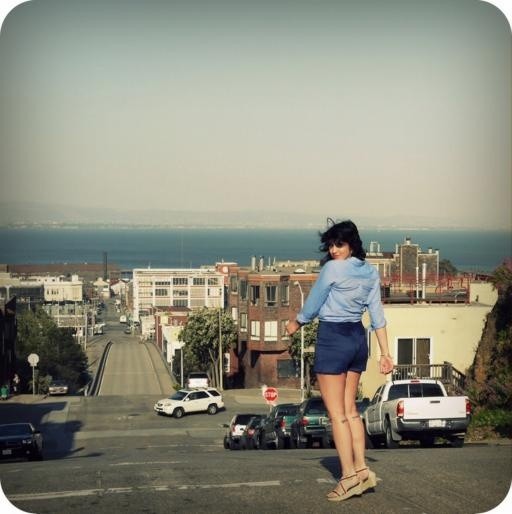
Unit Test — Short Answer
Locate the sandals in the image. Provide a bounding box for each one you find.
[326,468,378,503]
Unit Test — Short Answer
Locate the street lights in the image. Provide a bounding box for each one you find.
[293,281,304,401]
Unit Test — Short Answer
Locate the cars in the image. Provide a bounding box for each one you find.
[224,379,472,449]
[124,328,131,333]
[0,422,45,460]
[153,372,225,418]
[48,379,69,395]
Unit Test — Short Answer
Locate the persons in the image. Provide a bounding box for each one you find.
[285,220,394,502]
[0,373,20,400]
